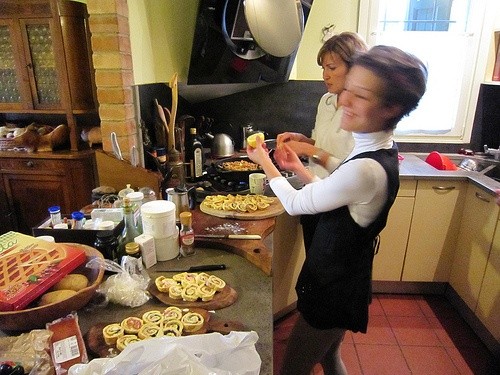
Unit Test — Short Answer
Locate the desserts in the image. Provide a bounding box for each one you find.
[155,271,225,301]
[204,194,276,213]
[103,306,204,352]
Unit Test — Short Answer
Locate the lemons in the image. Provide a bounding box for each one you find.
[247,133,264,148]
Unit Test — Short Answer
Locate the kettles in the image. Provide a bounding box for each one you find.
[205,131,236,158]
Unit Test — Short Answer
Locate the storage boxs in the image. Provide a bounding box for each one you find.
[32,213,125,239]
[0,231,87,312]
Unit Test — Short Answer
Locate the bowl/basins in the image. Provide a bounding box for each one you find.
[0,242,104,332]
[425,150,456,171]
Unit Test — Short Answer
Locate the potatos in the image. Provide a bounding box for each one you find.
[39,290,78,306]
[53,274,89,290]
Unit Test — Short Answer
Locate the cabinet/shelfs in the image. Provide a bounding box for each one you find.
[401,179,468,294]
[273,211,307,321]
[0,0,98,114]
[447,182,500,355]
[371,179,417,293]
[0,154,98,236]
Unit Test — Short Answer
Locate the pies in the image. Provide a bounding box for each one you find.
[0,247,66,292]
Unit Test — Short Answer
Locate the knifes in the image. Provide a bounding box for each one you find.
[156,263,226,273]
[195,235,262,240]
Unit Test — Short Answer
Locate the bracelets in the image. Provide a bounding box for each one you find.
[312,155,320,164]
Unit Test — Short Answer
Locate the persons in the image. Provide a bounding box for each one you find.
[274,30,369,311]
[246,45,428,375]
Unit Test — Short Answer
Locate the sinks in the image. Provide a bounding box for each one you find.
[414,153,498,175]
[481,163,500,195]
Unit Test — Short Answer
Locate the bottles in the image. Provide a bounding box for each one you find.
[179,211,197,257]
[116,202,143,262]
[71,212,85,230]
[93,231,123,274]
[123,242,143,275]
[186,128,203,178]
[48,205,62,226]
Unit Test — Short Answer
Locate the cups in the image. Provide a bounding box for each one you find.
[150,227,179,260]
[249,173,267,195]
[139,199,176,240]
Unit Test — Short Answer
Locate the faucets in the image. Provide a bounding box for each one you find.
[484,147,500,160]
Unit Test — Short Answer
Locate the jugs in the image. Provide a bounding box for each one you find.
[167,184,194,227]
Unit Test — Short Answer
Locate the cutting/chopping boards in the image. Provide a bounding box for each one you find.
[185,206,278,275]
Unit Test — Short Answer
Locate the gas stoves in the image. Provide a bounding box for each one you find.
[190,168,295,199]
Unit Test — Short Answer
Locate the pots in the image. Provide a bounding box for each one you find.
[212,155,280,181]
